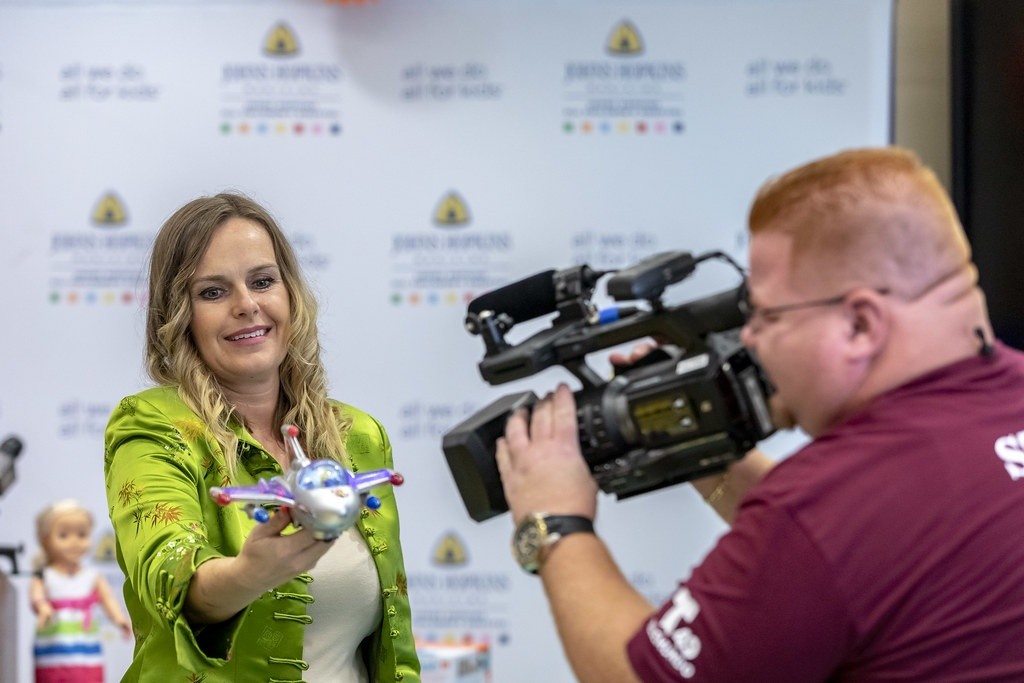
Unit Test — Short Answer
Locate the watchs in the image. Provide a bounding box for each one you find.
[511,512,594,574]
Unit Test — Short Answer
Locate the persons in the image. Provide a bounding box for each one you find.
[29,499,130,682]
[104,190,422,682]
[494,144,1022,681]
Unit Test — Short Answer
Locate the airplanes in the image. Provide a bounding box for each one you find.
[207,423,405,542]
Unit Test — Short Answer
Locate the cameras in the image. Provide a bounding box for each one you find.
[443,249,782,522]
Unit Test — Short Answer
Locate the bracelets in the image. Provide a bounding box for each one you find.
[705,461,734,504]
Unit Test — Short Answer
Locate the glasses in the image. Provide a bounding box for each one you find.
[739,287,892,334]
